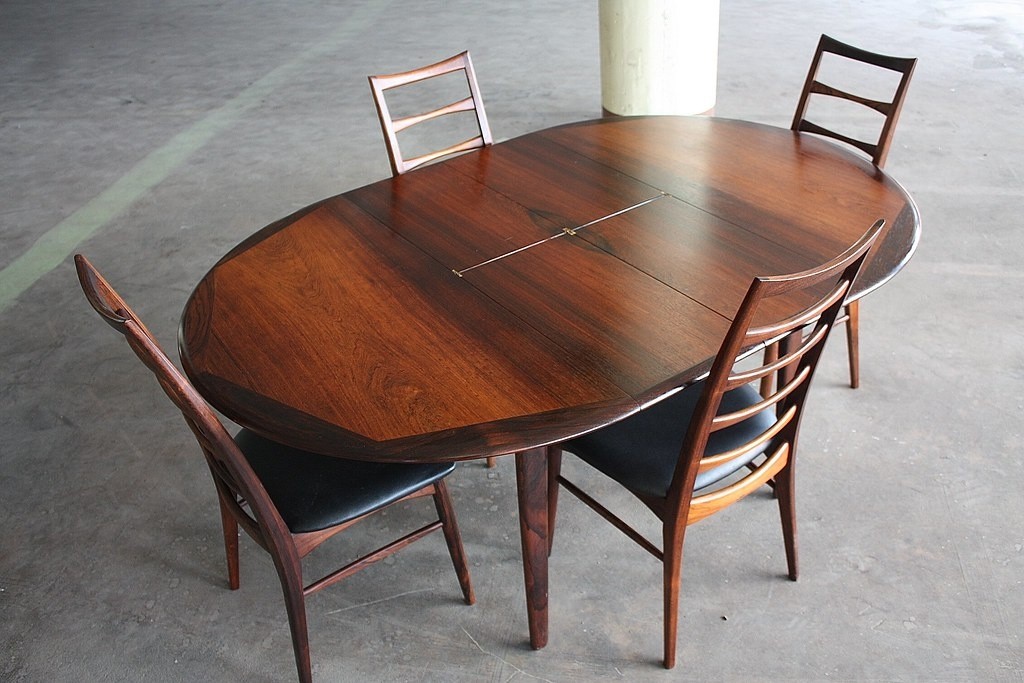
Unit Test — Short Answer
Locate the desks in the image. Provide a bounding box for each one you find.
[178,113,919,652]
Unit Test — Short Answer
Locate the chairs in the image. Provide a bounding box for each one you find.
[366,48,503,468]
[546,219,888,670]
[759,34,919,401]
[74,253,476,683]
